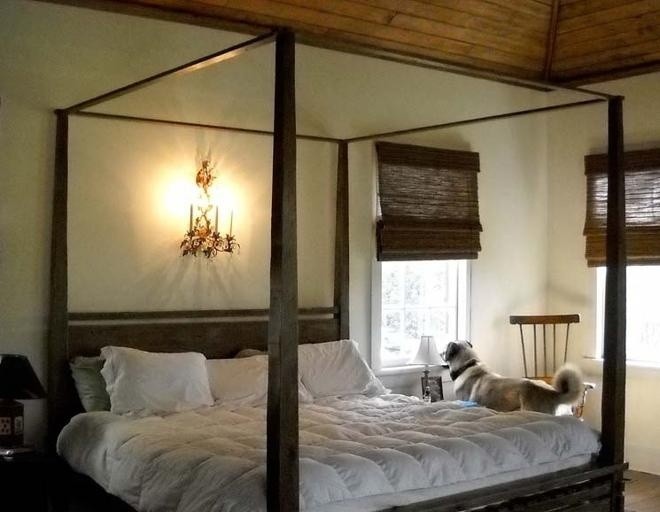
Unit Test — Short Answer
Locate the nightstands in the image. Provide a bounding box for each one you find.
[0,443,47,512]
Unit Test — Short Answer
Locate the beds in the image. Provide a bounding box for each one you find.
[49,306,629,509]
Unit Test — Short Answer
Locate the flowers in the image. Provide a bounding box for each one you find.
[179,230,240,262]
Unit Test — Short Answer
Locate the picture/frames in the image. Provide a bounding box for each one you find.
[421,376,444,402]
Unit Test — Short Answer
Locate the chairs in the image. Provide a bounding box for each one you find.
[509,314,596,419]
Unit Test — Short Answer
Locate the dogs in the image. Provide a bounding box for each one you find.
[438,339,585,416]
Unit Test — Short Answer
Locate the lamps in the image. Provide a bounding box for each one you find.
[0,355,49,449]
[407,336,449,403]
